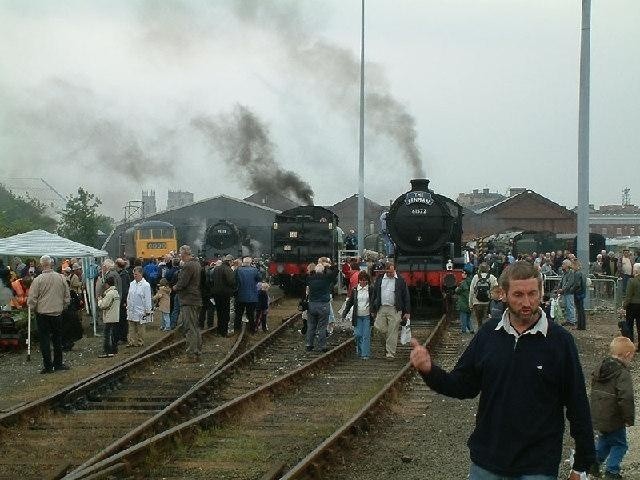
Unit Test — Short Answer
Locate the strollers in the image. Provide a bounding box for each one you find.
[540,289,563,321]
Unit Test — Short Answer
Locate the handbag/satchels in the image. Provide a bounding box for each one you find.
[400,317,411,345]
[298,294,308,311]
[618,313,634,342]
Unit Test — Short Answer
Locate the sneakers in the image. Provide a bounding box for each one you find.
[54,364,69,370]
[40,368,54,374]
[385,352,394,358]
[178,357,196,363]
[605,471,624,480]
[98,353,107,358]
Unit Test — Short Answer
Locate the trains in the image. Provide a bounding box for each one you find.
[268,205,341,293]
[121,220,178,269]
[198,218,255,267]
[365,179,464,313]
[466,230,569,265]
[0,306,37,352]
[557,232,608,261]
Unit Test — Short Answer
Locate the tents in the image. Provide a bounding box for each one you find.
[0,229,108,335]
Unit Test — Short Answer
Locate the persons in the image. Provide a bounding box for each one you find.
[96,245,271,359]
[455,247,588,331]
[592,250,640,295]
[409,258,599,480]
[27,251,72,374]
[0,256,82,306]
[298,254,409,361]
[621,263,640,352]
[588,335,636,480]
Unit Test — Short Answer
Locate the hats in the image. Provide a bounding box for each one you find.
[105,277,114,286]
[563,259,571,267]
[223,254,233,261]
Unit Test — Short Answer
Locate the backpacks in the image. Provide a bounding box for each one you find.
[473,273,492,302]
